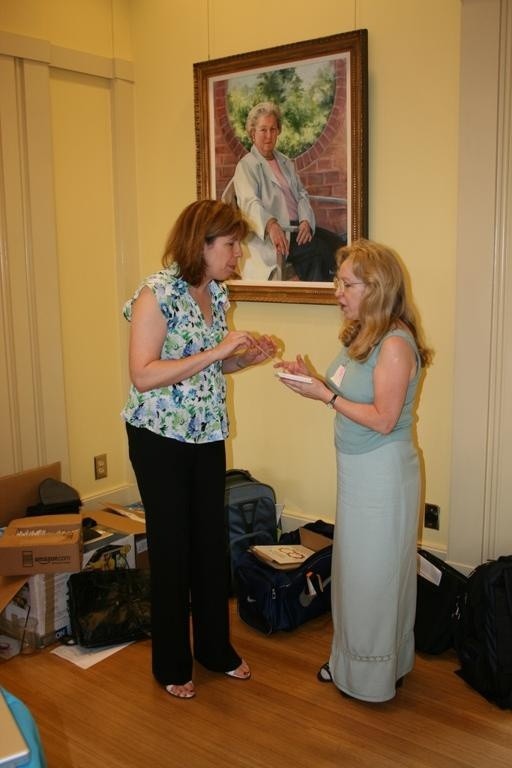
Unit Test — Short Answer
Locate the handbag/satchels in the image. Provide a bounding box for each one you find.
[68,568,152,649]
[414,549,468,654]
[234,521,333,637]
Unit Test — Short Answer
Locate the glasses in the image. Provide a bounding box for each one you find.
[333,277,363,292]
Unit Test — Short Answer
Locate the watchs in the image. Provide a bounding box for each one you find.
[325,394,339,407]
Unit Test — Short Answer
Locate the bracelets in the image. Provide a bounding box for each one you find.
[236,355,246,369]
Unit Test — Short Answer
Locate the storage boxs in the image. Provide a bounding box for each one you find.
[0,461,151,651]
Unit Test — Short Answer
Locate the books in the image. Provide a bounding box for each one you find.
[0,692,31,767]
[248,544,318,571]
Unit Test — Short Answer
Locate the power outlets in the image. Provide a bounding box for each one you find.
[95,454,108,480]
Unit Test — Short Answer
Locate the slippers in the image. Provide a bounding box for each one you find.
[227,661,252,681]
[317,662,332,682]
[165,681,195,697]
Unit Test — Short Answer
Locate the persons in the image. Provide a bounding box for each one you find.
[273,238,435,704]
[233,102,347,281]
[119,199,279,700]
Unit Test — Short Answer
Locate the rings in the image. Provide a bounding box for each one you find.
[276,244,280,248]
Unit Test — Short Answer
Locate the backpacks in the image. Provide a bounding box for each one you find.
[225,468,278,596]
[454,555,512,711]
